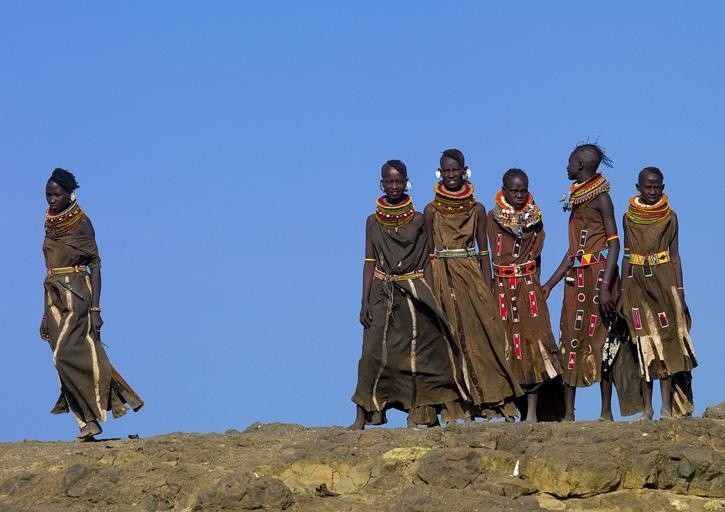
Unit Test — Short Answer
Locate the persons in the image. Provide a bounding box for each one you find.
[615,166,700,422]
[39,167,144,442]
[422,149,524,426]
[540,141,620,423]
[486,168,563,424]
[345,158,461,430]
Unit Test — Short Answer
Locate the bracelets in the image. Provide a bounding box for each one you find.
[89,307,101,312]
[676,286,684,290]
[601,278,610,286]
